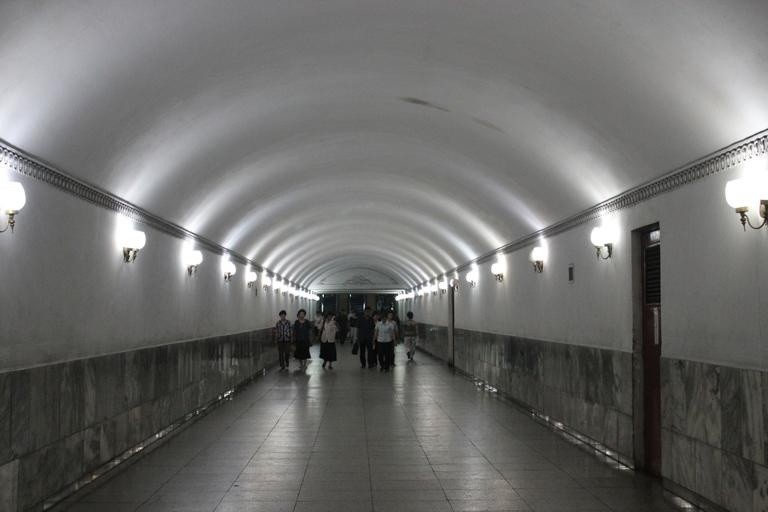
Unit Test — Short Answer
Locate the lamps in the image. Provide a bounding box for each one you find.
[245,270,257,288]
[220,259,236,282]
[393,267,478,302]
[528,244,550,274]
[263,276,320,304]
[0,181,27,234]
[723,179,767,232]
[490,262,504,282]
[589,226,612,261]
[121,229,146,263]
[186,249,203,276]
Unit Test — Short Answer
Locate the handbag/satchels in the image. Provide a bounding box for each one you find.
[352,342,357,355]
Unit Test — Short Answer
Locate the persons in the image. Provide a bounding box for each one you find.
[274,294,421,374]
